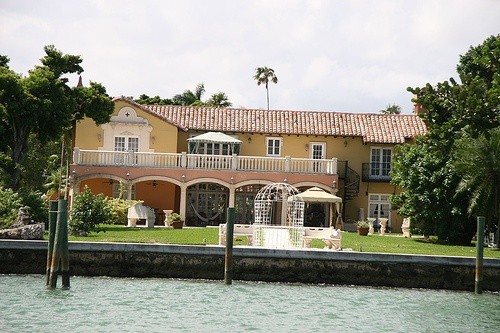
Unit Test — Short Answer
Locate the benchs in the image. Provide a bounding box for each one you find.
[218,223,342,251]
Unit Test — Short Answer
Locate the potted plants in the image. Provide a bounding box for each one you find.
[354,219,370,236]
[167,213,184,230]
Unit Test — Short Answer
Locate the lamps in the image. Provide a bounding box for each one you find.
[230,175,235,184]
[332,178,337,188]
[151,136,156,145]
[284,176,288,184]
[182,173,186,182]
[97,134,102,142]
[126,171,131,180]
[305,143,309,152]
[72,168,76,177]
[247,136,252,144]
[151,181,158,189]
[343,140,348,147]
